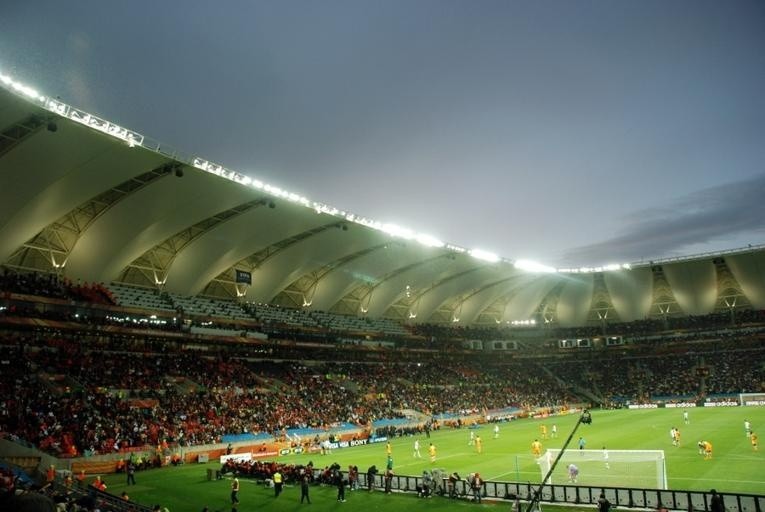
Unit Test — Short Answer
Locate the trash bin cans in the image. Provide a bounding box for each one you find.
[208,470,216,482]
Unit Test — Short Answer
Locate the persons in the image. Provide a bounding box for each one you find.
[1,264,239,512]
[239,307,765,511]
